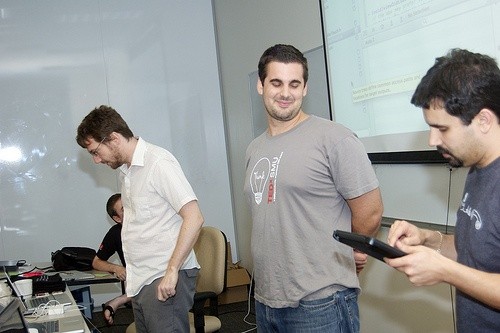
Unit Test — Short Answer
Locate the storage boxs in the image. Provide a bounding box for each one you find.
[205,265,253,306]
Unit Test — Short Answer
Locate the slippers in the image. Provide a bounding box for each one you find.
[101,303,114,324]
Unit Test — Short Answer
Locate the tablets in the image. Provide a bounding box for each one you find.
[333,229,405,263]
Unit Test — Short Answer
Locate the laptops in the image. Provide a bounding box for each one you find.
[3,265,74,315]
[0,298,85,333]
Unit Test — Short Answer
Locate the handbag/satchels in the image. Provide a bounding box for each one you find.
[51,247,96,272]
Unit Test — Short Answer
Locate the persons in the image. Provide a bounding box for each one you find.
[92,194,131,323]
[76,105,204,333]
[388,47,500,333]
[242,44,385,333]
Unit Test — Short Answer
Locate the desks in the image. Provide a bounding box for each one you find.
[21,262,121,333]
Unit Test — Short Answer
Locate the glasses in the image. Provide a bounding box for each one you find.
[90,135,107,158]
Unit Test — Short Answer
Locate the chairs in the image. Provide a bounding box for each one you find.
[125,226,228,333]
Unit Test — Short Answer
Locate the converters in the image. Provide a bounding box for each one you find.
[48,305,64,315]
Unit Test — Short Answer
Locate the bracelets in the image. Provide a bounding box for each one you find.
[435,231,443,252]
